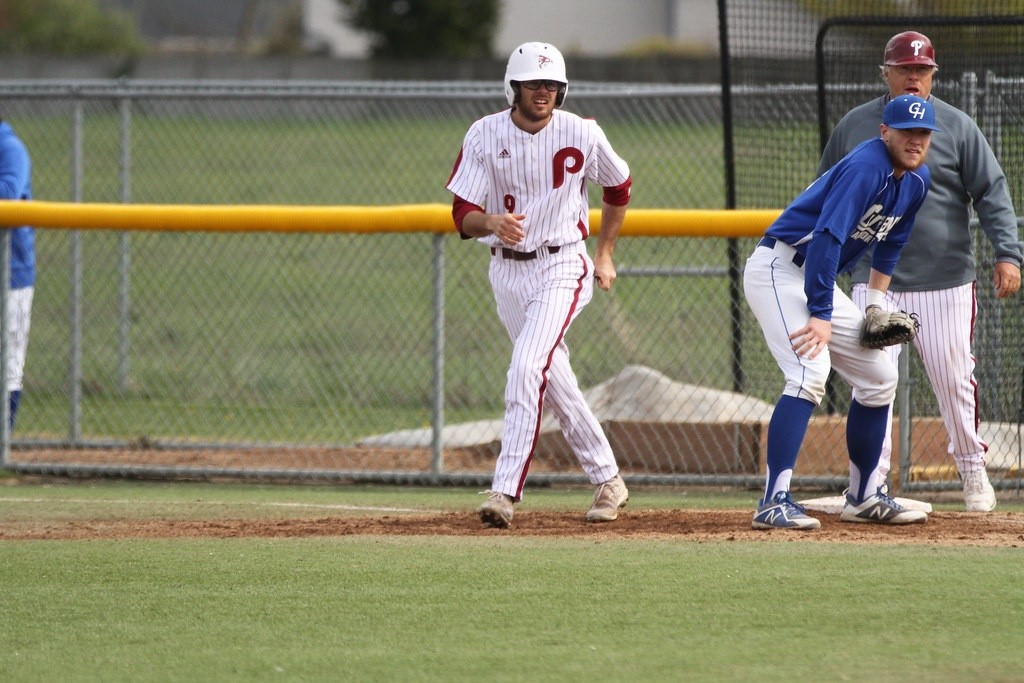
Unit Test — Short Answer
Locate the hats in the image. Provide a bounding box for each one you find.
[882,92,942,133]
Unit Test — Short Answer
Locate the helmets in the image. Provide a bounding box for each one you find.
[884,31,939,68]
[505,42,569,108]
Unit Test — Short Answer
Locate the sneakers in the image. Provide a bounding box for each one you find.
[479,492,515,528]
[586,474,630,522]
[751,492,822,530]
[959,468,997,512]
[840,484,929,525]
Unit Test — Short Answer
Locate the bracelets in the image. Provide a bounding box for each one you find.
[864,304,883,312]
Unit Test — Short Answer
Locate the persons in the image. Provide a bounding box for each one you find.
[816,30,1020,514]
[452,38,632,530]
[0,115,39,452]
[741,95,941,531]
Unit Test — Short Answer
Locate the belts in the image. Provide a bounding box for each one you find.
[490,242,560,261]
[759,236,806,268]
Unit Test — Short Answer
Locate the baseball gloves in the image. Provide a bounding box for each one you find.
[861,302,921,351]
